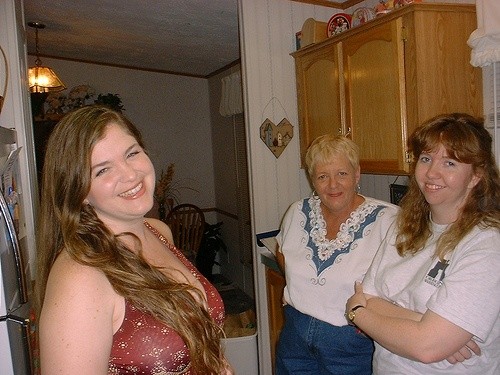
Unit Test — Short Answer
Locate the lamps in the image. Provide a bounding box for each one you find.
[27,21,67,93]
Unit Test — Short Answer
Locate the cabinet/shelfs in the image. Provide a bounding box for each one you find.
[289,2,483,177]
[264,268,285,375]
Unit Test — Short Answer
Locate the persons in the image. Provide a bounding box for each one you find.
[30,105,234,375]
[346,113,500,375]
[276,134,401,375]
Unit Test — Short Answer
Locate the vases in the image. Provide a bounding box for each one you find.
[160,202,165,221]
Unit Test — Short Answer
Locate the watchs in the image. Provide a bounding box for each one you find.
[348,306,363,324]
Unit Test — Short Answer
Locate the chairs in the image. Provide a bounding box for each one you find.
[166,204,206,266]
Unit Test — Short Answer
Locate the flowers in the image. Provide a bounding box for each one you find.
[34,84,125,121]
[154,163,200,205]
